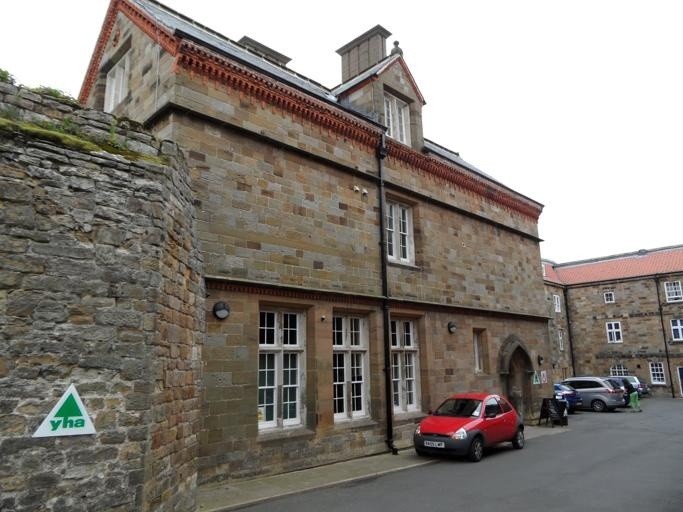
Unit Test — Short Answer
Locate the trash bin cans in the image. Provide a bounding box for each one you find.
[554,400,568,425]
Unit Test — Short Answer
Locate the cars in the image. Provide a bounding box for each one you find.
[608,376,650,397]
[552,383,583,413]
[608,377,630,407]
[560,377,627,413]
[412,392,525,463]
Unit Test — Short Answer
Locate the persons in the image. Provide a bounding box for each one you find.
[618,376,641,413]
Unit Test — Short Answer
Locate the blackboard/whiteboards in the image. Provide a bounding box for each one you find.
[540,398,548,419]
[543,398,561,420]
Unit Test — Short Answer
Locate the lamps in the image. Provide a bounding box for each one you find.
[448,321,457,333]
[212,301,230,320]
